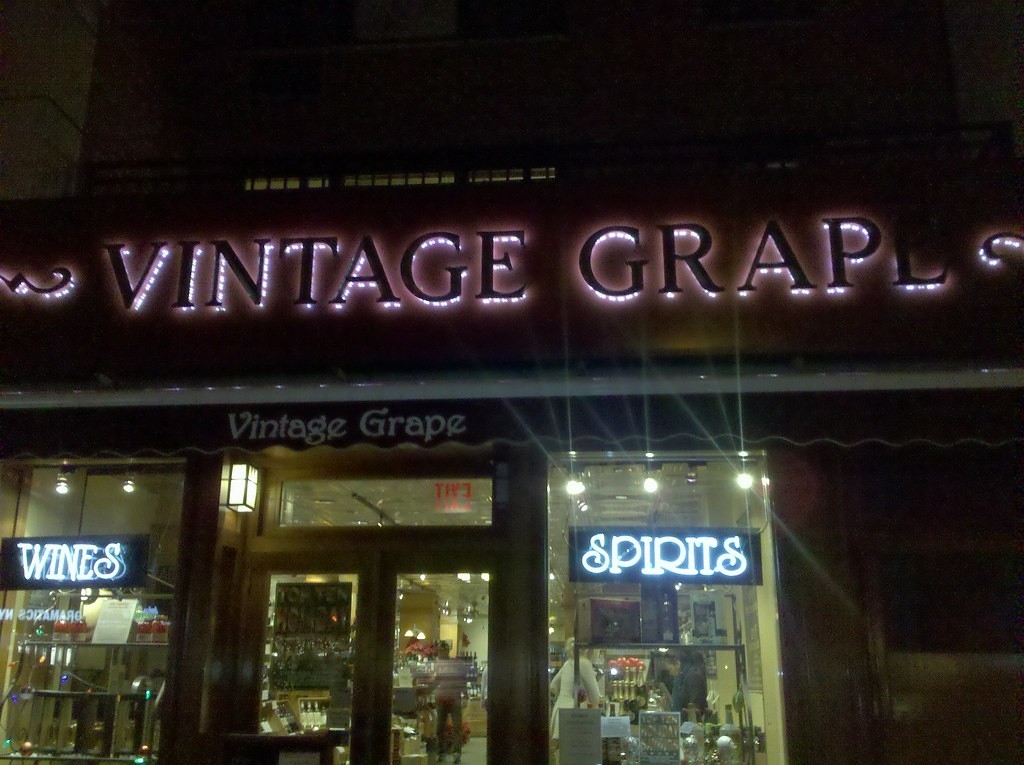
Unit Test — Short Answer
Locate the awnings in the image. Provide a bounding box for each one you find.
[1,364,1024,462]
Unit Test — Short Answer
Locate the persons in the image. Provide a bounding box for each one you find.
[416,641,469,765]
[549,638,600,754]
[481,665,488,711]
[672,648,708,722]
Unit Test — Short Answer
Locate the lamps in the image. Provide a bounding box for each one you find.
[123,478,134,492]
[228,462,259,513]
[55,474,69,494]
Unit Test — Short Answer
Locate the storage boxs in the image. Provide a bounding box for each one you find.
[262,700,306,736]
[294,696,330,729]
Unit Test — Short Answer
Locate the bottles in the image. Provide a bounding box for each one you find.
[686,702,744,765]
[300,700,327,728]
[610,666,650,725]
[455,651,481,701]
